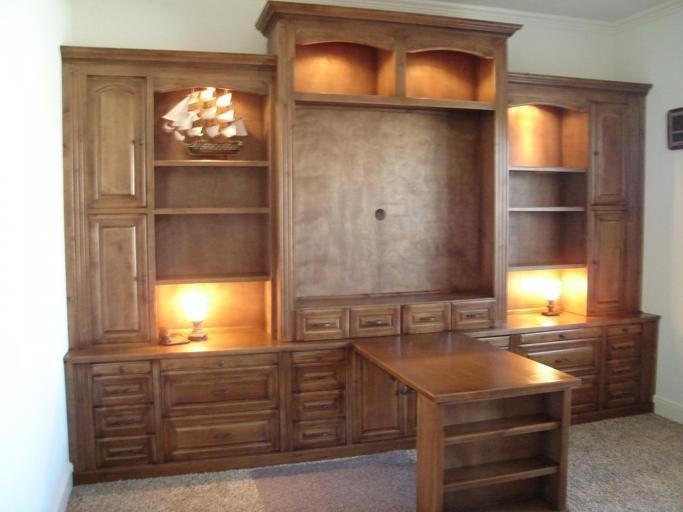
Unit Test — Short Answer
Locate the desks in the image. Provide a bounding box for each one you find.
[350,331,581,512]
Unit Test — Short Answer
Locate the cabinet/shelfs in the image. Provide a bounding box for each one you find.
[511,83,646,309]
[514,328,656,416]
[68,356,280,473]
[298,310,401,452]
[63,59,269,336]
[289,40,485,297]
[403,302,501,332]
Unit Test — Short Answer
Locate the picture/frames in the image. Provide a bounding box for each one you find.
[667,107,683,150]
